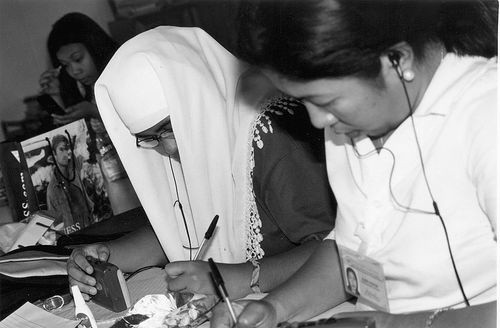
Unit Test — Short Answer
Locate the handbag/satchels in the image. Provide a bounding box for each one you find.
[1,116,113,238]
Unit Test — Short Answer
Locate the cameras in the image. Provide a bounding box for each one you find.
[82,256,131,313]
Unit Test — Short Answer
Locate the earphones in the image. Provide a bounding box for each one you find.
[389,51,401,66]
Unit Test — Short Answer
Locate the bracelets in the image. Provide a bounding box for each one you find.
[425,306,457,328]
[246,259,263,294]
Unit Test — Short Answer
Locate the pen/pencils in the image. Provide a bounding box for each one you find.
[207,257,238,325]
[193,214,220,261]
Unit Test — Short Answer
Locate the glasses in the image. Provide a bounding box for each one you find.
[136,128,173,148]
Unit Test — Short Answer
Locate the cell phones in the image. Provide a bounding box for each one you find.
[38,94,66,116]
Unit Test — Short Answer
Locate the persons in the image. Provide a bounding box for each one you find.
[211,0,498,328]
[25,12,120,140]
[46,135,95,236]
[66,26,337,311]
